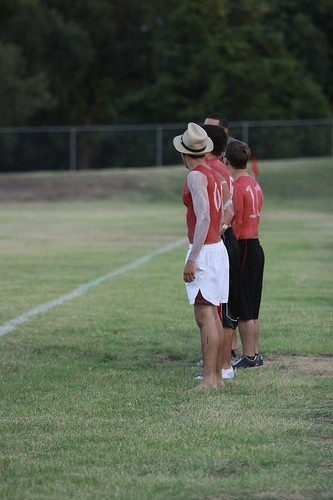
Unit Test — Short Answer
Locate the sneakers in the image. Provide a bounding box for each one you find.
[193,368,237,380]
[232,354,263,370]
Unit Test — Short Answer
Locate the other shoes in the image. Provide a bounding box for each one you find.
[231,350,242,365]
[197,359,203,366]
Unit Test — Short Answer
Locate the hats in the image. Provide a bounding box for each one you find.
[172,122,214,156]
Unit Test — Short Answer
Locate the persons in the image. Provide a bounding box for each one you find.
[196,124,263,380]
[172,122,230,393]
